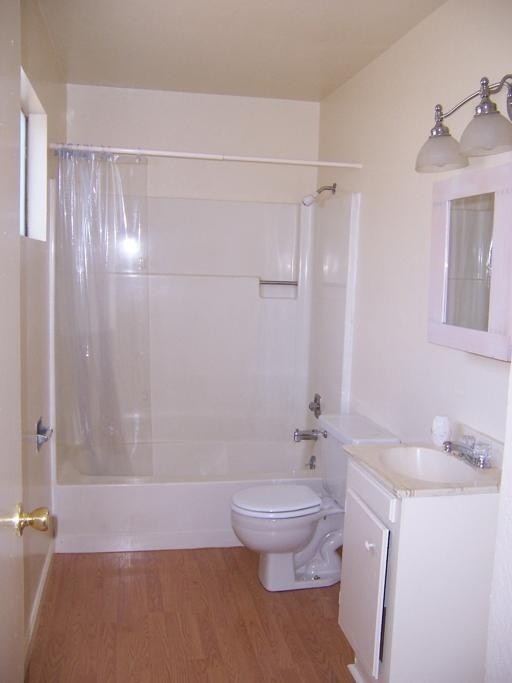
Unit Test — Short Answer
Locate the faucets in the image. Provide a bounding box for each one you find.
[443,439,475,462]
[293,428,318,441]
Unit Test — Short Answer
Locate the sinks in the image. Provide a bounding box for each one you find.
[379,445,477,483]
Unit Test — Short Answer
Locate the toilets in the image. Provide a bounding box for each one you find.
[230,410,400,593]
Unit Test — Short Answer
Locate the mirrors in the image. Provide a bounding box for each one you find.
[444,190,496,332]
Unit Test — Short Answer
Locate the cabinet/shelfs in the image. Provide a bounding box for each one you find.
[336,446,507,681]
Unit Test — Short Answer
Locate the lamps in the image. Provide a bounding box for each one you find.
[414,70,512,174]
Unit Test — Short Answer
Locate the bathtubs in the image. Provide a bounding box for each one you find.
[57,437,326,555]
